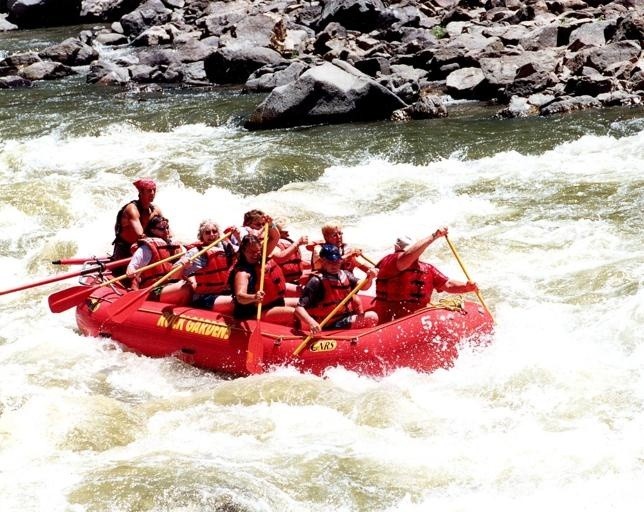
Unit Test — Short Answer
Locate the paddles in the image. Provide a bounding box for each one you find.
[104,229,234,323]
[48,251,186,314]
[245,215,269,374]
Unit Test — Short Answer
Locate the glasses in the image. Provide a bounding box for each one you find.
[252,222,266,226]
[154,226,169,231]
[332,232,343,239]
[205,230,218,234]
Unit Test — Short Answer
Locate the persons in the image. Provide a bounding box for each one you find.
[292,222,379,333]
[110,178,187,301]
[173,210,309,325]
[373,224,479,323]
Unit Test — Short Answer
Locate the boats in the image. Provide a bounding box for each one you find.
[74,251,493,381]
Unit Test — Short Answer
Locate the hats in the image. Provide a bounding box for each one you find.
[320,244,342,262]
